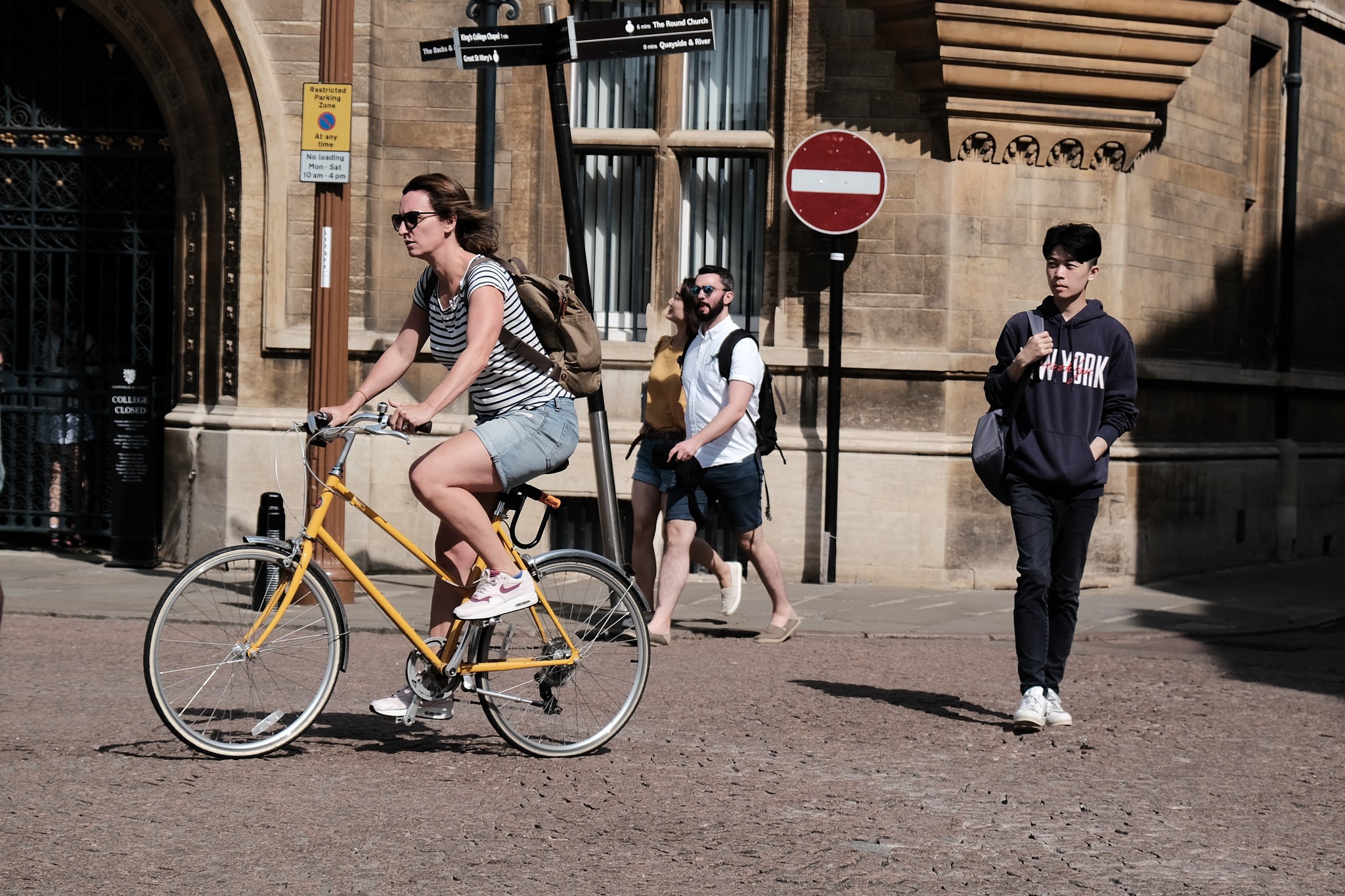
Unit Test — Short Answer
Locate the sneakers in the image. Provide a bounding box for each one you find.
[1047,688,1072,727]
[453,568,538,621]
[369,670,454,720]
[1012,687,1047,726]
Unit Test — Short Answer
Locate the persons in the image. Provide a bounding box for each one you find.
[984,222,1140,731]
[620,264,803,648]
[316,172,580,720]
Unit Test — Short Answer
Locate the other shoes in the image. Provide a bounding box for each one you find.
[622,612,655,628]
[753,607,801,643]
[720,561,742,616]
[622,627,671,646]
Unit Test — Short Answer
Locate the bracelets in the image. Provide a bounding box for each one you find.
[356,390,367,402]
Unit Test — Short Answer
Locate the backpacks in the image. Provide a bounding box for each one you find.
[464,257,602,398]
[676,328,786,522]
[971,310,1044,508]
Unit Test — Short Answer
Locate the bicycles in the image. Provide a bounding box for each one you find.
[143,402,654,758]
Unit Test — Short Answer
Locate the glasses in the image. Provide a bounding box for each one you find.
[690,286,726,297]
[391,211,439,232]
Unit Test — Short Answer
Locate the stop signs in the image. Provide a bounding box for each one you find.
[784,131,888,234]
